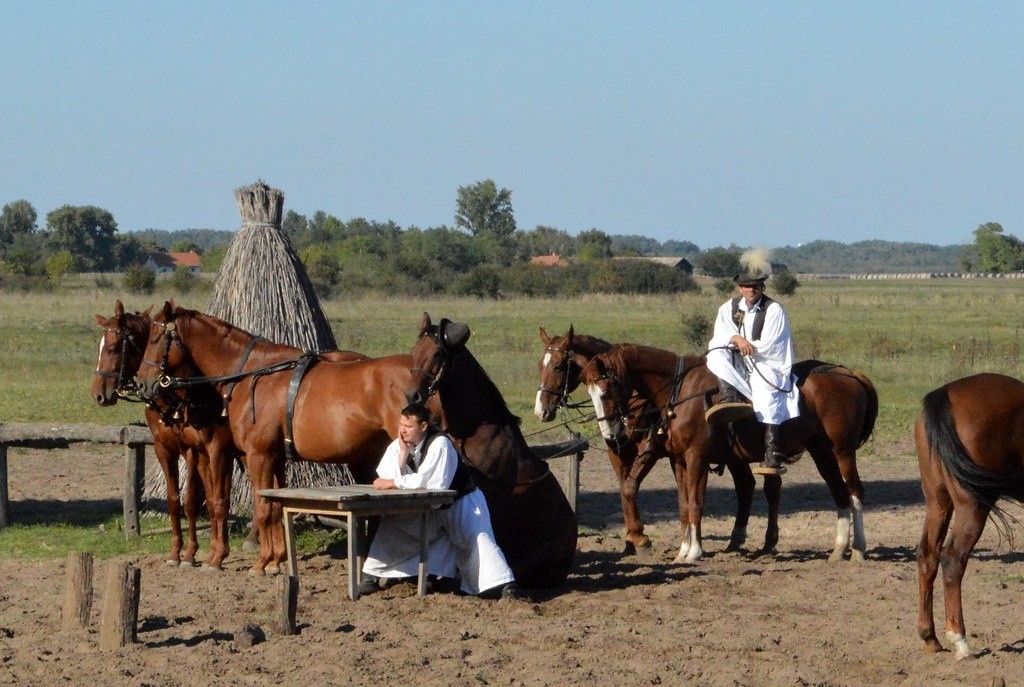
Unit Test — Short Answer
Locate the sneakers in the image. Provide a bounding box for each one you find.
[500,582,521,600]
[356,571,380,596]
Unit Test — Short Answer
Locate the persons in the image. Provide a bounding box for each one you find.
[707,247,800,469]
[357,403,515,596]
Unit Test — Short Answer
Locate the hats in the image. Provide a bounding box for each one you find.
[733,247,775,287]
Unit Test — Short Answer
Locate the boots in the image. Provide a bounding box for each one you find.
[703,378,753,424]
[751,423,787,475]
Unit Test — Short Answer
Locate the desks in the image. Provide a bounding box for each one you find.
[255,483,458,601]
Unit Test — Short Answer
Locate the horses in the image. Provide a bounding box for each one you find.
[402,310,580,601]
[90,296,446,579]
[912,371,1023,663]
[531,322,880,566]
